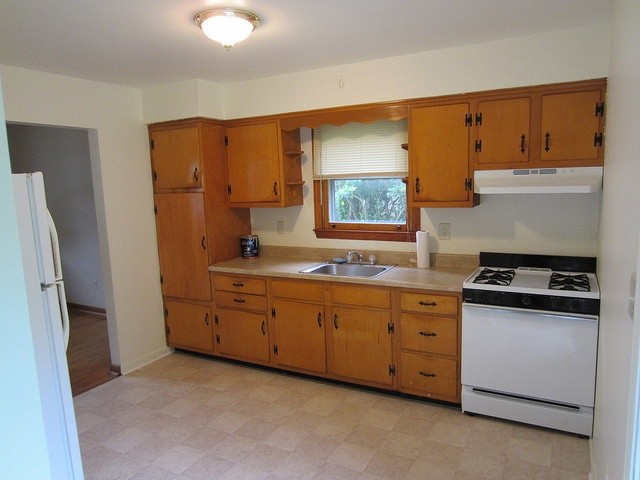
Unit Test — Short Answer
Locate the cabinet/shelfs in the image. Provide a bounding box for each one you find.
[145,117,228,191]
[209,272,272,370]
[474,85,607,169]
[394,287,462,405]
[163,304,214,356]
[408,99,479,208]
[151,191,251,304]
[224,118,306,208]
[269,277,396,391]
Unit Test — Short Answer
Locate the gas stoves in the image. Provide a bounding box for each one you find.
[463,265,600,318]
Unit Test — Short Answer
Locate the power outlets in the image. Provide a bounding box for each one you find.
[439,223,450,240]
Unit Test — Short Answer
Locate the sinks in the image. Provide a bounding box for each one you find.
[298,263,399,279]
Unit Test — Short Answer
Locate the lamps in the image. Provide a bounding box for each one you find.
[193,7,261,52]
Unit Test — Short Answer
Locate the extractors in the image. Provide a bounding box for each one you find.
[473,166,604,195]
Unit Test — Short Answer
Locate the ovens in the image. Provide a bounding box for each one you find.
[461,302,599,439]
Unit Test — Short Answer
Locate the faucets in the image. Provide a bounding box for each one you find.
[348,251,363,262]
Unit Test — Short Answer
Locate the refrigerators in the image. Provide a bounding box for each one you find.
[0,171,87,480]
